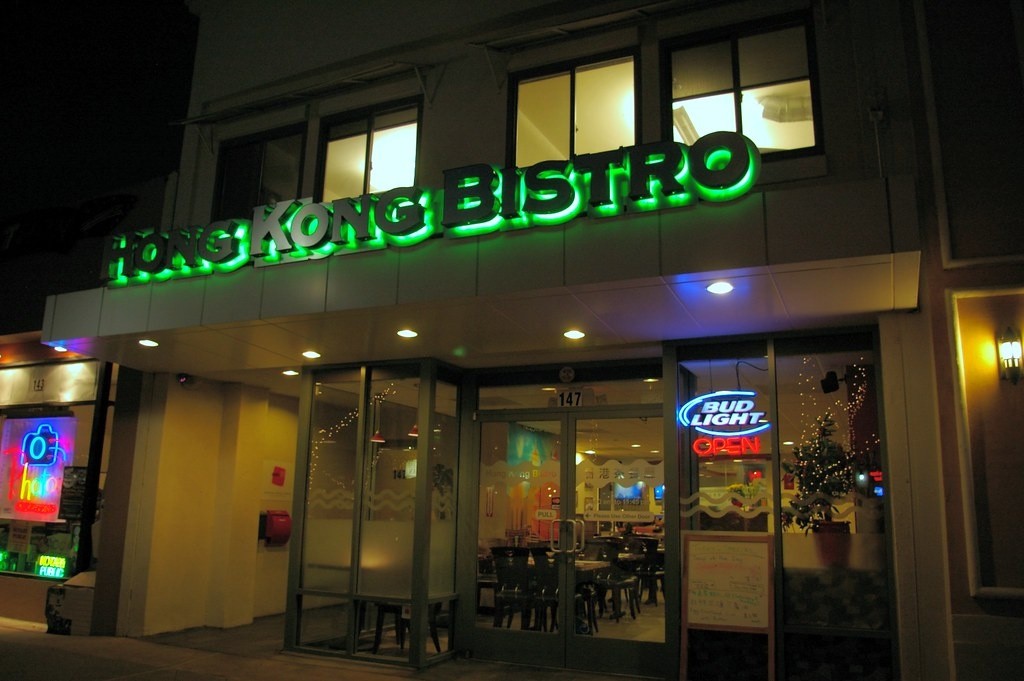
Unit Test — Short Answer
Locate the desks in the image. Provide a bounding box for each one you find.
[510,559,610,636]
[577,553,645,622]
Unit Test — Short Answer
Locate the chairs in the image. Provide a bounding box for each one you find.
[372,602,441,655]
[491,535,665,636]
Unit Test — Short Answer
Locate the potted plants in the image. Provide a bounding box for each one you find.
[782,412,853,568]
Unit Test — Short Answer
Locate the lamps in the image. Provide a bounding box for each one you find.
[673,105,698,146]
[409,384,420,437]
[996,327,1024,385]
[369,393,385,443]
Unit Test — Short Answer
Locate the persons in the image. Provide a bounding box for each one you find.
[653,516,664,529]
